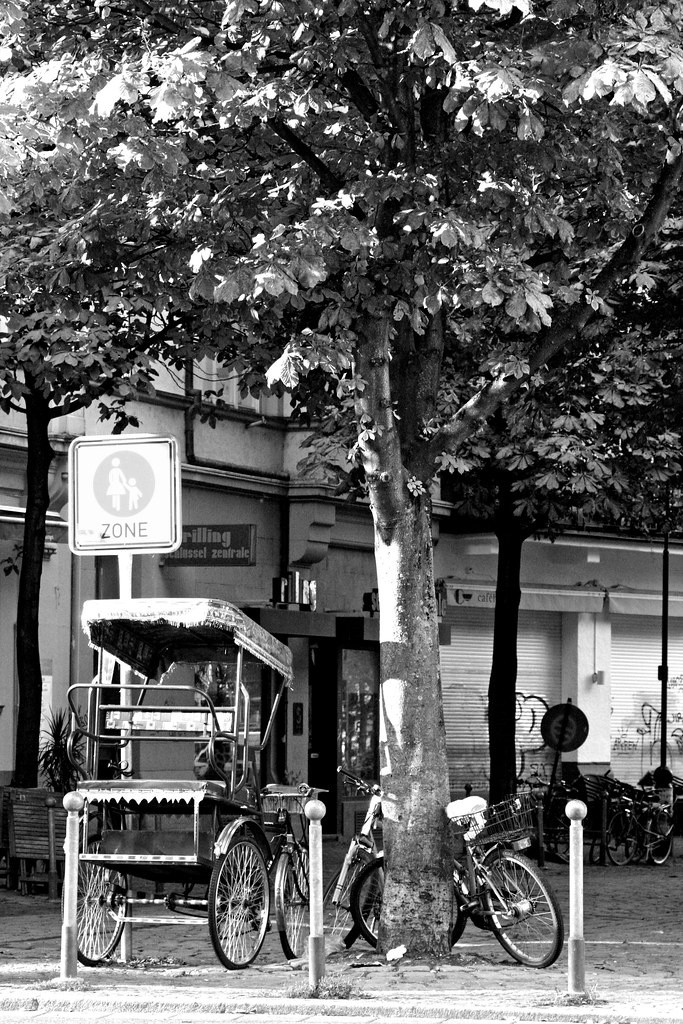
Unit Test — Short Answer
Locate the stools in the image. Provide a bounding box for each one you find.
[77,779,259,816]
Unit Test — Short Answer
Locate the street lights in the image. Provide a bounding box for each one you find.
[637,473,683,835]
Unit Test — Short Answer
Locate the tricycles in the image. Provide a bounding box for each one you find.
[62,597,329,970]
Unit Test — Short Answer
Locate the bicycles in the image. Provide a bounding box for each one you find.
[323,765,566,968]
[587,781,675,867]
